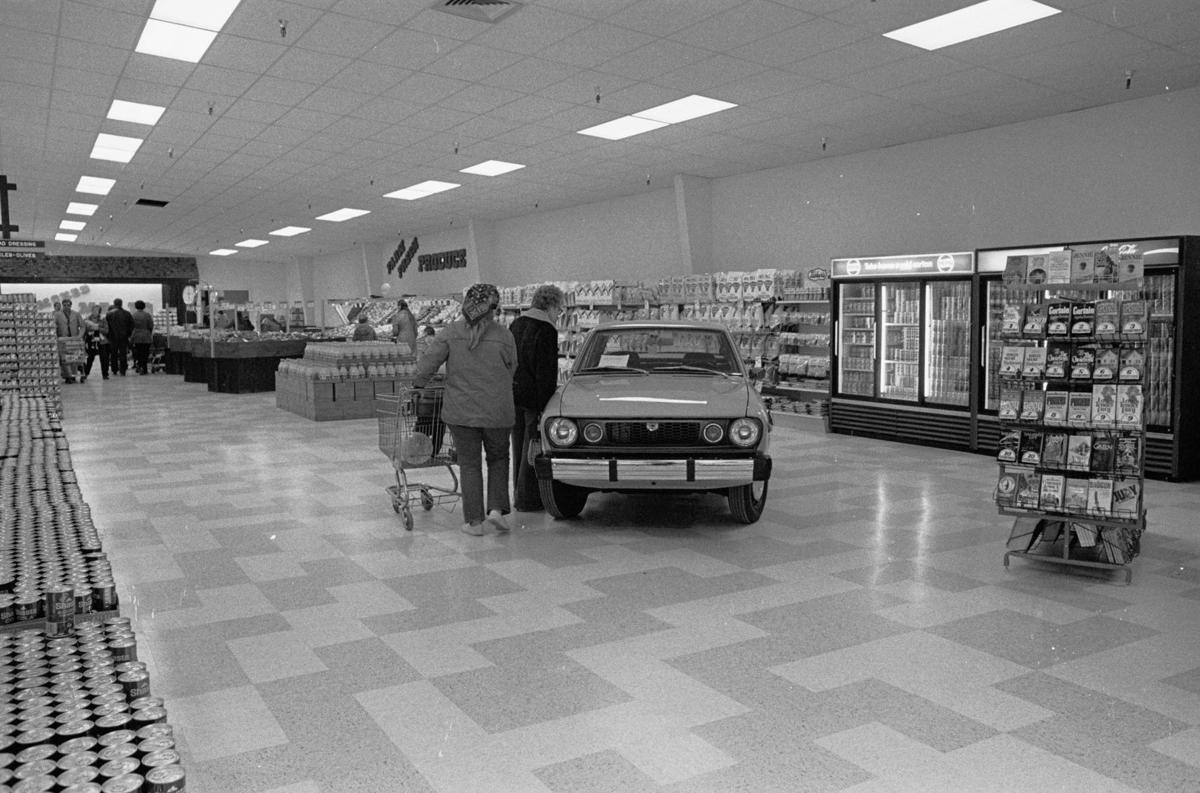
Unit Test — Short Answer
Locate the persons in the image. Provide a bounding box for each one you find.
[422,327,436,351]
[509,284,565,510]
[353,315,378,342]
[409,282,518,535]
[54,297,154,383]
[391,298,418,351]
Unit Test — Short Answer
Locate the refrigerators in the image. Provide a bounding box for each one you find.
[828,251,974,453]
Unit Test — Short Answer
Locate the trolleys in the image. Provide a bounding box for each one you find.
[56,333,88,385]
[374,380,465,535]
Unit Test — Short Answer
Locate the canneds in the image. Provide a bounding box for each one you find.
[0,391,187,793]
[986,281,1038,410]
[842,282,969,406]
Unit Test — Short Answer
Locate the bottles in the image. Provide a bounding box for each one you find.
[278,340,448,380]
[842,280,876,397]
[879,280,921,402]
[925,280,972,406]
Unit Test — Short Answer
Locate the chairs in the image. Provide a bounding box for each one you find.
[610,350,640,369]
[683,352,716,369]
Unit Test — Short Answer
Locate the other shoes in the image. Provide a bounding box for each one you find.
[462,521,483,536]
[517,505,544,512]
[113,370,117,374]
[102,375,110,379]
[488,509,510,530]
[121,369,126,375]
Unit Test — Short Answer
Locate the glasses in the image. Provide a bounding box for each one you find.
[555,304,563,315]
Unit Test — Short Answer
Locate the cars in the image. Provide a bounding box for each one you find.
[526,318,776,524]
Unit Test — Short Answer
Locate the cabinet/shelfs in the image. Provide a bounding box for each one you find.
[992,282,1155,589]
[501,301,572,386]
[662,283,801,425]
[571,291,662,371]
[768,267,832,433]
[351,292,464,328]
